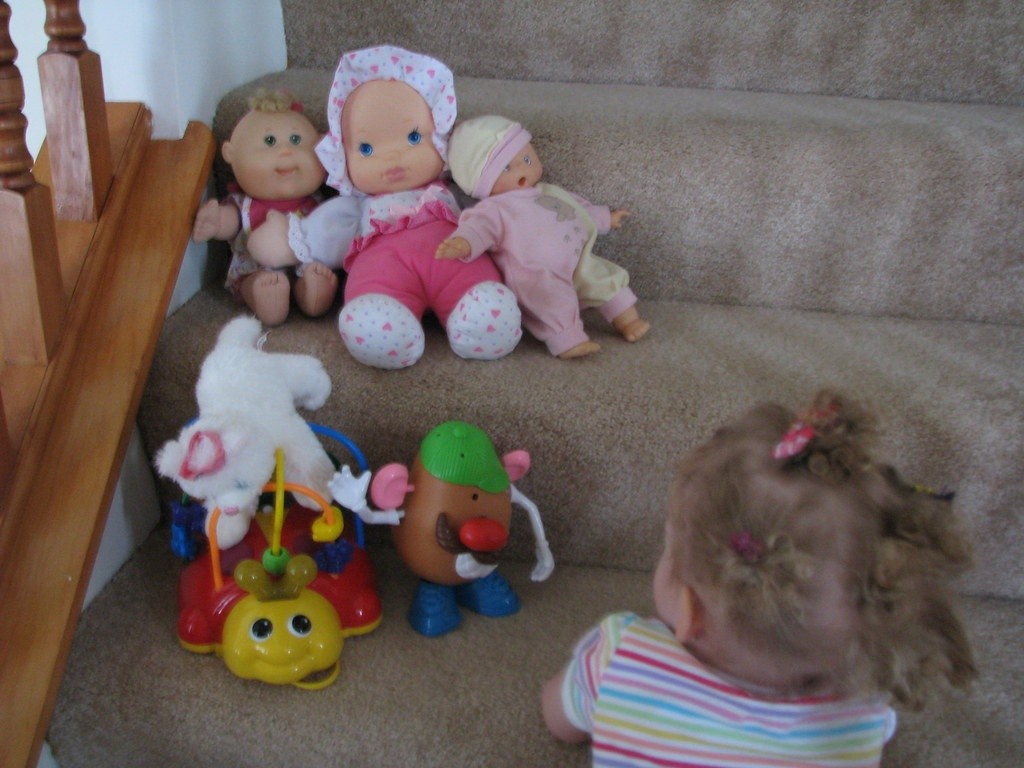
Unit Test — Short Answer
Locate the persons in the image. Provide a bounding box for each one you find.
[540,390,977,768]
[194,45,650,368]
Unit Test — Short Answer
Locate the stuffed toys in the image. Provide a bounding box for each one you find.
[153,317,338,550]
